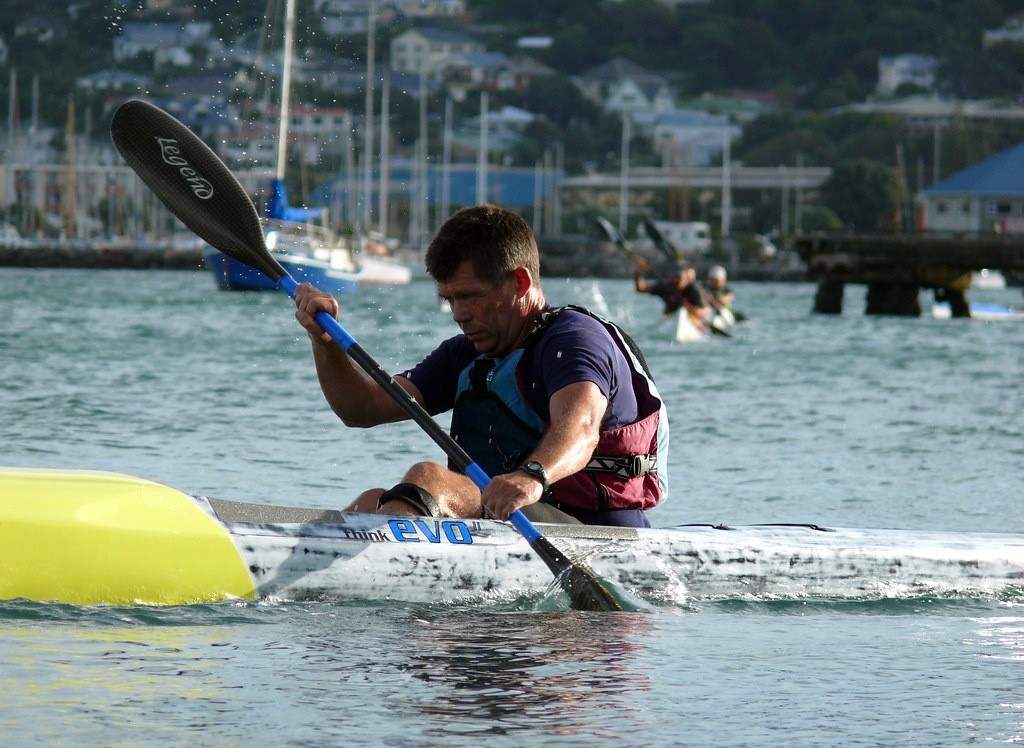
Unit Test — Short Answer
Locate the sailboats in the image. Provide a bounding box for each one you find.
[1,0,647,287]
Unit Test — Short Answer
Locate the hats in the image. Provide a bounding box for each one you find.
[707,264,726,280]
[665,259,687,276]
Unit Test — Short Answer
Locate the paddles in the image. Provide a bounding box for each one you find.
[109,98,619,612]
[594,215,749,338]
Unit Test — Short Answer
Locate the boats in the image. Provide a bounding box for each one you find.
[656,302,745,344]
[1,458,1024,621]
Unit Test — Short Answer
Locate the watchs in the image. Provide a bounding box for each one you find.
[518,461,549,493]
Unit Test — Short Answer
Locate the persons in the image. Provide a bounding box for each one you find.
[630,255,710,320]
[297,206,667,527]
[704,266,733,313]
